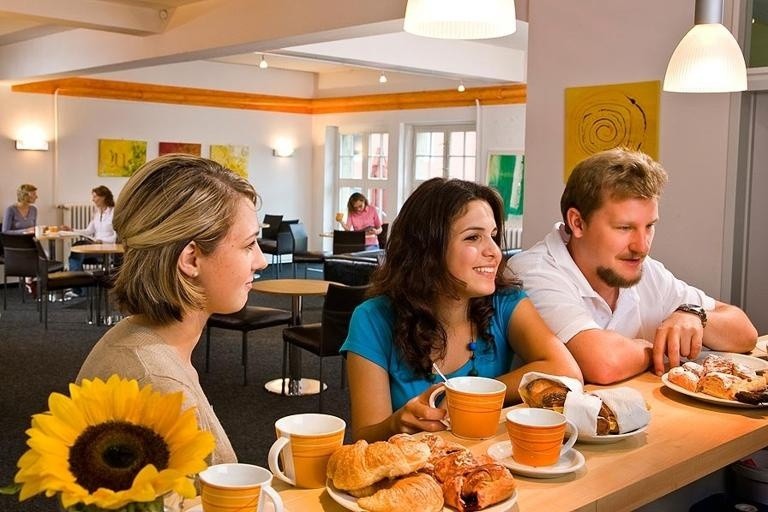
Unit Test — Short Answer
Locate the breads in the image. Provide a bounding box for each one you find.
[665,352,767,407]
[523,375,652,434]
[327,430,516,512]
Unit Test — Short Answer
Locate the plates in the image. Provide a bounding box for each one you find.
[571,422,653,449]
[755,335,767,352]
[486,440,586,478]
[659,351,768,410]
[323,475,521,512]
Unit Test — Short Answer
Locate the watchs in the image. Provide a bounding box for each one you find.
[674,303,707,329]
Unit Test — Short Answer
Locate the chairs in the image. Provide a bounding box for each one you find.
[0,223,123,330]
[205,305,292,386]
[282,283,372,412]
[256,213,389,279]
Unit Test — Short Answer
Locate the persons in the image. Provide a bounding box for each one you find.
[65,185,119,299]
[339,178,585,444]
[75,153,267,510]
[338,192,382,253]
[3,185,38,299]
[502,147,758,387]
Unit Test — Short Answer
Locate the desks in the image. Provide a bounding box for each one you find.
[251,279,336,397]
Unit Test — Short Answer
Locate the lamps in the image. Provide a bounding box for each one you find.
[15,140,48,151]
[272,148,293,157]
[402,0,516,40]
[662,1,748,94]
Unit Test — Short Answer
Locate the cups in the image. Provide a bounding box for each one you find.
[268,412,347,489]
[506,407,580,469]
[336,213,343,222]
[197,462,286,511]
[48,225,58,233]
[427,375,508,440]
[34,225,44,241]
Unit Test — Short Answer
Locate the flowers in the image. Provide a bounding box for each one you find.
[0,371,217,512]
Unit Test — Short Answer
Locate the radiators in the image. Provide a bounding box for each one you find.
[63,204,109,270]
[500,229,521,250]
